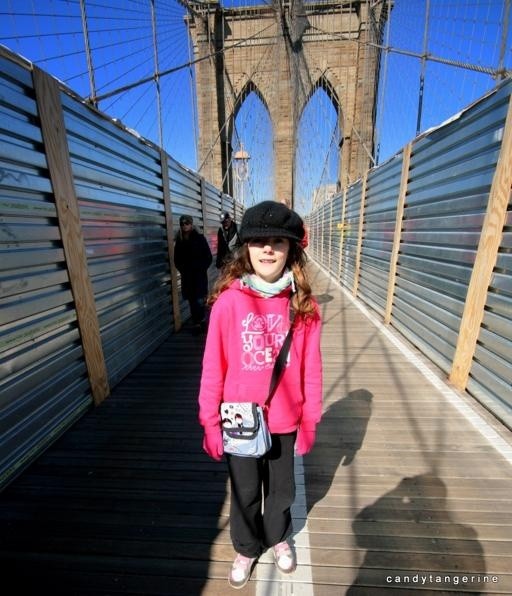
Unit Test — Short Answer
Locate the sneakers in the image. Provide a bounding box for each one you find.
[272,540,297,573]
[227,553,257,590]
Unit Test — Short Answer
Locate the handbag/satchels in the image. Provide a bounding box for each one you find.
[219,401,274,459]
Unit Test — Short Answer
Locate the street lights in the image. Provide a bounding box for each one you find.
[234,142,252,203]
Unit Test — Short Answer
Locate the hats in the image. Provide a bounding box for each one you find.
[219,211,230,223]
[239,199,307,243]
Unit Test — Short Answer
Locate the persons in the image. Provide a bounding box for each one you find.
[194,201,325,591]
[213,210,241,270]
[173,214,213,329]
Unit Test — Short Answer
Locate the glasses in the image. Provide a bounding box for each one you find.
[180,221,189,227]
